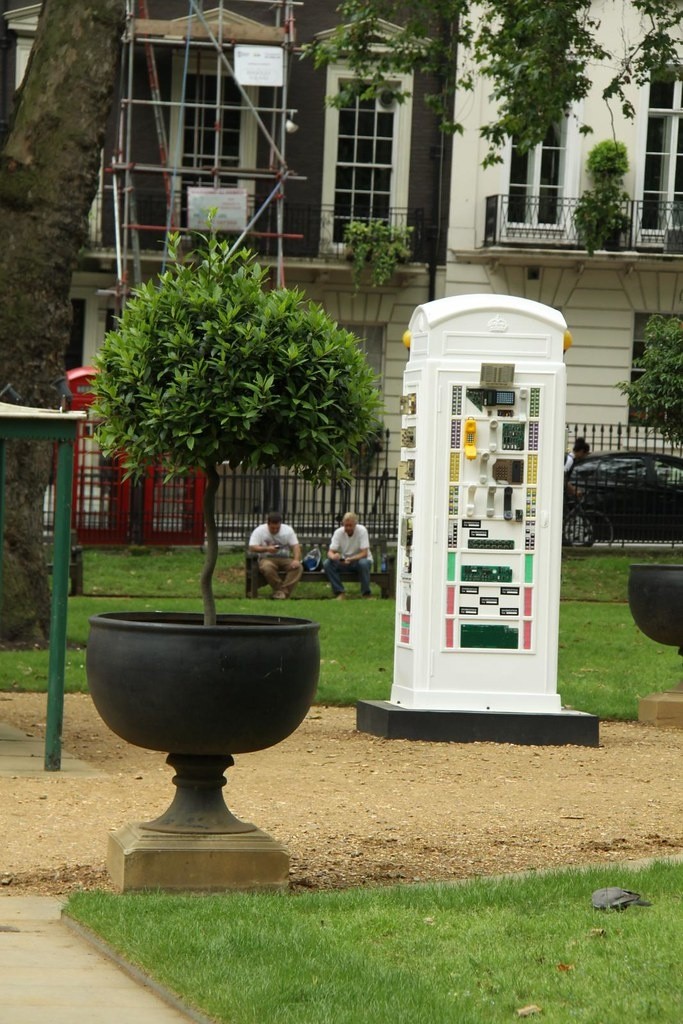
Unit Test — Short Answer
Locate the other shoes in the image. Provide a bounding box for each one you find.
[274,591,286,599]
[363,594,373,600]
[336,593,345,600]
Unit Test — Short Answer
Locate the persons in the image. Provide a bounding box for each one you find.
[323,511,373,600]
[564,444,589,498]
[249,512,303,599]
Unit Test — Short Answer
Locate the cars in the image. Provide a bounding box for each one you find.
[565,450,683,543]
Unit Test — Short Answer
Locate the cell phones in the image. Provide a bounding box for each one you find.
[340,558,345,561]
[275,545,282,550]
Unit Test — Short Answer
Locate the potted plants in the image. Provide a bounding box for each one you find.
[341,219,415,297]
[86,206,386,835]
[615,314,683,696]
[572,139,631,257]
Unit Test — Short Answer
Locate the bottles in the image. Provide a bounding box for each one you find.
[382,554,386,571]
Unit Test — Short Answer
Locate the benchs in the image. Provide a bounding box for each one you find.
[246,538,390,600]
[45,544,83,597]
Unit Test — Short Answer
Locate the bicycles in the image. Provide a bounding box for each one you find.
[565,465,613,547]
